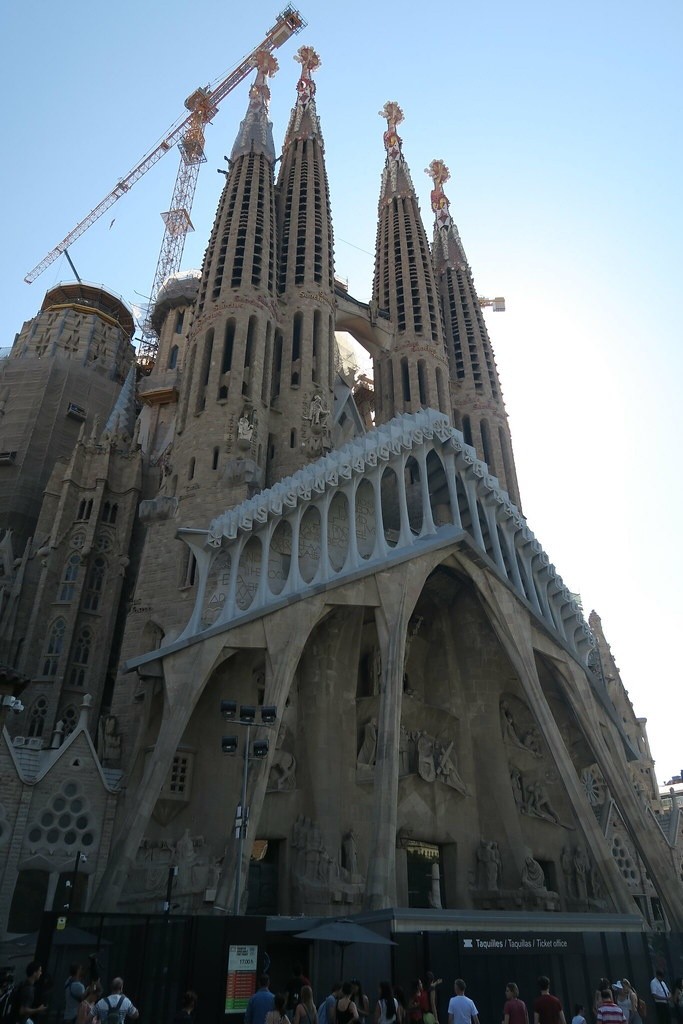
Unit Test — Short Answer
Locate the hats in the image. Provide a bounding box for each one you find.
[611,981,623,991]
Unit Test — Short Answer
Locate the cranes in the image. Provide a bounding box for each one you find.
[21,1,308,373]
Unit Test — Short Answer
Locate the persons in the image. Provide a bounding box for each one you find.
[170,828,203,912]
[245,970,444,1024]
[0,960,139,1024]
[571,977,642,1024]
[309,394,331,428]
[533,975,565,1024]
[500,982,529,1024]
[358,717,378,764]
[291,812,329,879]
[237,410,253,446]
[561,845,600,900]
[512,767,561,823]
[343,829,358,873]
[399,722,461,775]
[447,979,480,1024]
[521,856,544,893]
[650,969,683,1024]
[476,839,502,889]
[501,700,544,755]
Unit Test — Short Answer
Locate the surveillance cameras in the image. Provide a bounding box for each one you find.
[12,704,25,714]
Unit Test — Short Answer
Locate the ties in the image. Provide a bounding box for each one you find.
[660,982,667,997]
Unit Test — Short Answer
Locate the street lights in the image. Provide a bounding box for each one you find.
[218,702,280,919]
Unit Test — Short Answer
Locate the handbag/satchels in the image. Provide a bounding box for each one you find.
[667,1001,675,1008]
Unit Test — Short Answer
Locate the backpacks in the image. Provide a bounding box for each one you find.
[630,993,646,1018]
[103,994,125,1024]
